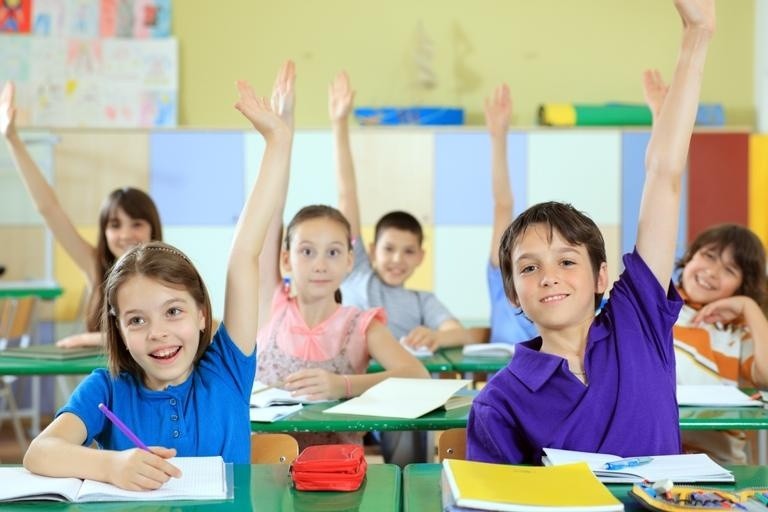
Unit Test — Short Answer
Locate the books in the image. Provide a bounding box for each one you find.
[245,373,330,407]
[539,445,736,491]
[663,379,762,413]
[0,452,240,511]
[435,455,628,512]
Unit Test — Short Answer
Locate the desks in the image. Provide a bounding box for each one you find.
[246,388,478,464]
[1,461,404,510]
[440,344,514,390]
[677,388,766,465]
[366,346,452,383]
[1,343,110,375]
[401,460,767,511]
[1,283,65,348]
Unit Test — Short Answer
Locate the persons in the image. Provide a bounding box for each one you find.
[327,69,467,469]
[2,74,165,352]
[253,54,434,403]
[478,79,549,344]
[642,67,768,466]
[19,78,294,493]
[460,1,719,467]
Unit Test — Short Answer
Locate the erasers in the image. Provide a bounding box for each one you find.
[652,478,674,495]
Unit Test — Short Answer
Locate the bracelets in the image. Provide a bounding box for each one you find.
[340,373,354,400]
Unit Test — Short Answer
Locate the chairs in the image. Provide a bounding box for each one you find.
[1,297,38,453]
[438,427,466,464]
[250,433,298,465]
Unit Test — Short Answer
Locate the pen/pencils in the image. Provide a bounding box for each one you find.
[665,490,768,512]
[98,403,152,453]
[604,457,654,470]
